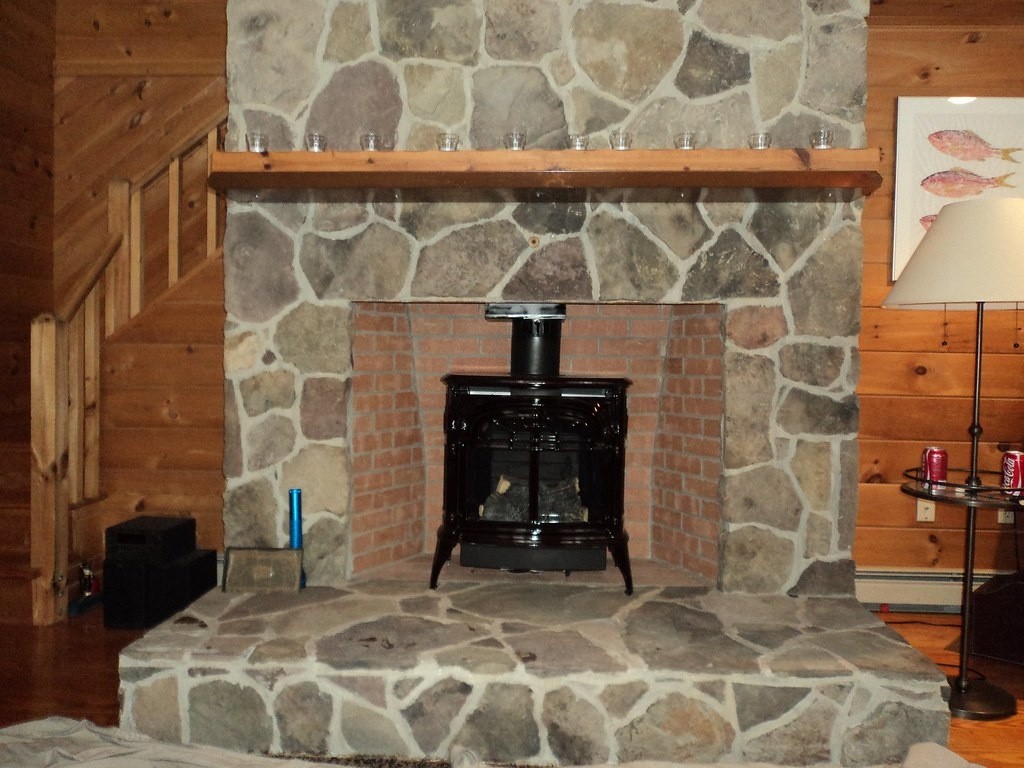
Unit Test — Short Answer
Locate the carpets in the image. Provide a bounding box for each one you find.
[0,716,457,768]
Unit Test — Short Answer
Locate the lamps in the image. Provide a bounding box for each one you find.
[877,196,1024,721]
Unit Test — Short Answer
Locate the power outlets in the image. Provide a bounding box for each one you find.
[998,508,1015,524]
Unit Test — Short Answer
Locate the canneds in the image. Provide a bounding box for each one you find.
[1002,450,1024,496]
[920,446,947,492]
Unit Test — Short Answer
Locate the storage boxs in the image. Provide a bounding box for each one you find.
[102,516,217,628]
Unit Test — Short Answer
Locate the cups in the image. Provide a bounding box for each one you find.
[246,132,270,152]
[609,131,634,151]
[673,132,696,149]
[360,133,383,152]
[503,132,526,150]
[303,130,328,152]
[748,132,772,149]
[565,134,590,150]
[435,133,459,151]
[809,131,834,149]
